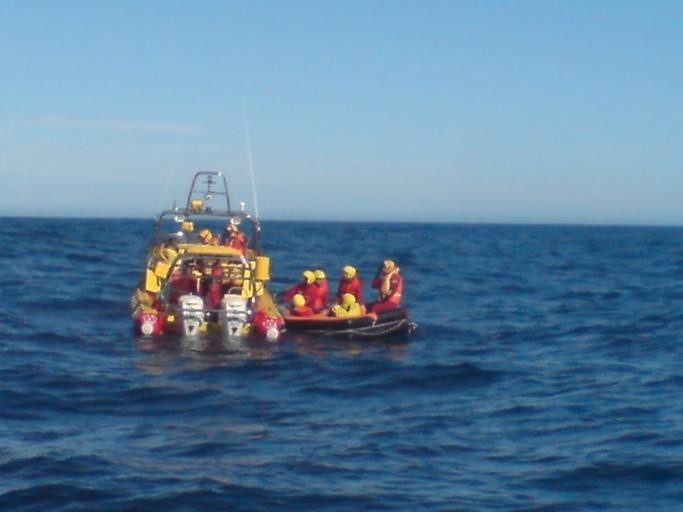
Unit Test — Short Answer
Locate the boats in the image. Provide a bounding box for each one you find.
[130,169,285,338]
[281,301,406,330]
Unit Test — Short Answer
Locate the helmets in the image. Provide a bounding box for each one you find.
[164,232,179,243]
[223,223,239,233]
[341,292,356,308]
[292,294,305,308]
[341,265,356,280]
[301,268,325,285]
[198,230,212,244]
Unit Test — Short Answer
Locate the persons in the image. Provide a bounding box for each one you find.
[167,224,403,318]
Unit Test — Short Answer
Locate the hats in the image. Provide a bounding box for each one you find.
[383,259,394,270]
